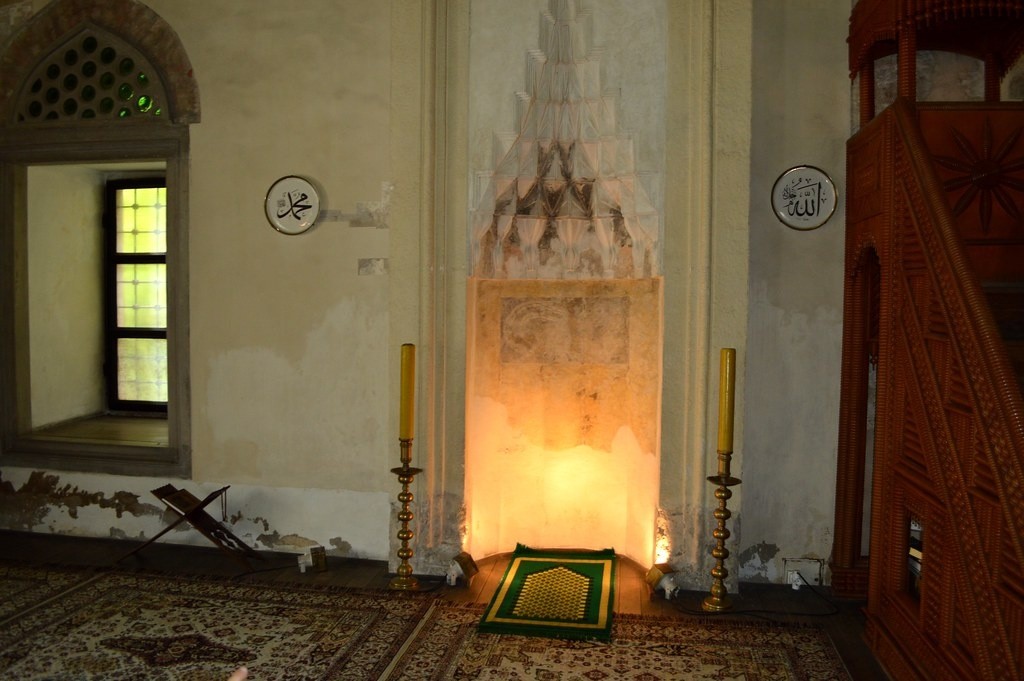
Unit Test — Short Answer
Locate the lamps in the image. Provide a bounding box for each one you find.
[445,552,479,586]
[645,564,684,600]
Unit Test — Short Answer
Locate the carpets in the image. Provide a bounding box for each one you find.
[476,543,617,642]
[0,557,858,681]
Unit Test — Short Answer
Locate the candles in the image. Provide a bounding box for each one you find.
[719,348,736,452]
[399,343,415,441]
